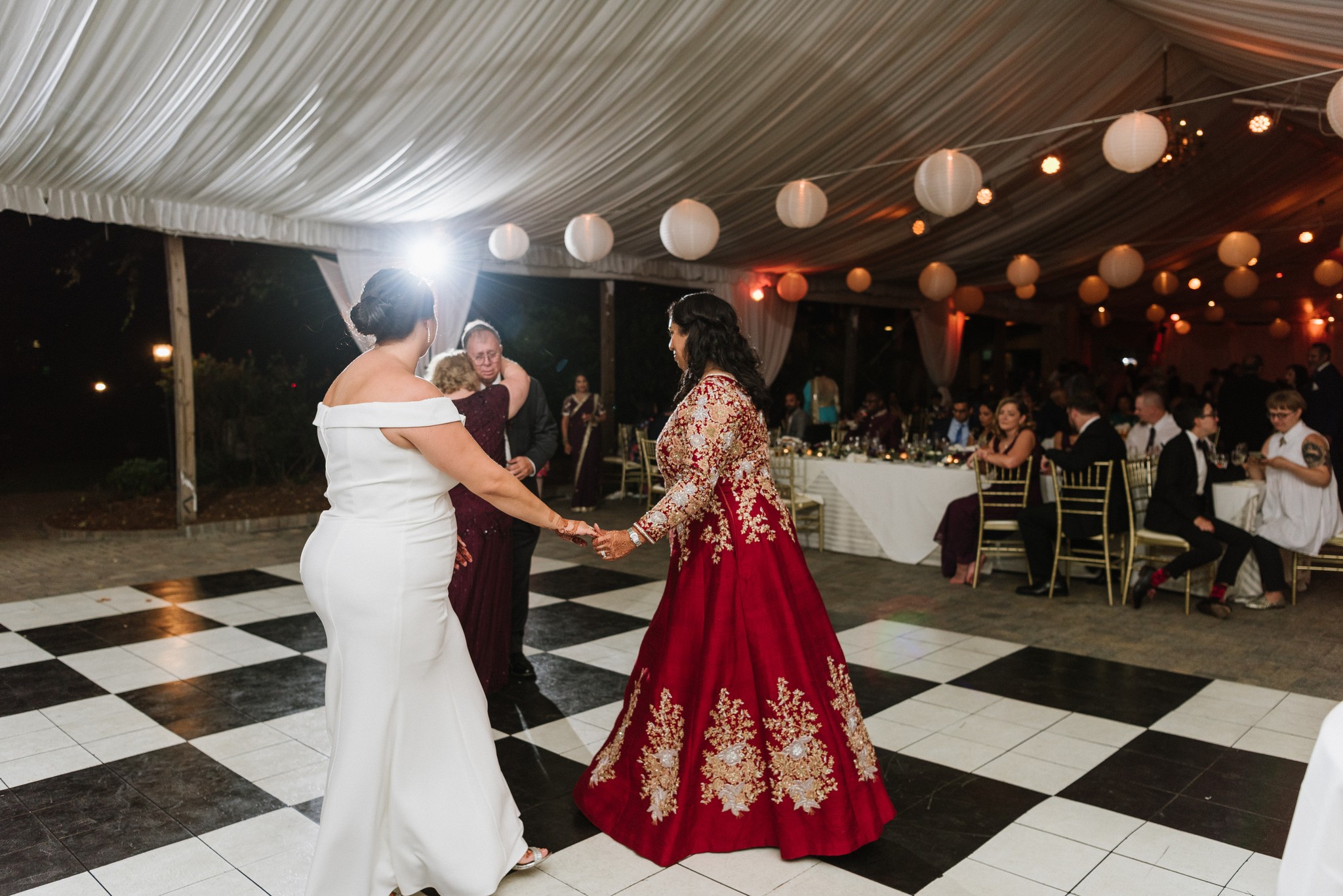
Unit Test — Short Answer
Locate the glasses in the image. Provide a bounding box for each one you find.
[1196,409,1219,418]
[1268,412,1293,419]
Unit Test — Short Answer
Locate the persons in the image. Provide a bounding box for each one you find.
[571,290,896,868]
[1243,389,1343,608]
[300,267,600,895]
[1127,398,1252,618]
[426,349,531,692]
[561,373,606,511]
[629,342,1343,507]
[932,397,1040,585]
[459,320,560,680]
[1015,392,1137,597]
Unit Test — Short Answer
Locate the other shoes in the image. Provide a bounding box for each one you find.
[1243,594,1285,609]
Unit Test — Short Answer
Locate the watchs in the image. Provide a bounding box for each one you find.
[627,527,642,548]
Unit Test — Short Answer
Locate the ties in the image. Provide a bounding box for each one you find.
[1146,428,1155,454]
[954,423,964,444]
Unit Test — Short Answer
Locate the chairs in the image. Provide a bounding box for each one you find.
[603,408,1343,616]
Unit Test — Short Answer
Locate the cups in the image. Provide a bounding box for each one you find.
[1212,453,1228,468]
[1231,451,1246,466]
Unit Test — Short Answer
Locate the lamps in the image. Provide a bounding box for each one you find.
[475,32,1343,346]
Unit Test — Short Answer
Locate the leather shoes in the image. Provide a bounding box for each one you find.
[1015,578,1068,596]
[1196,597,1231,618]
[509,652,535,676]
[1132,565,1157,609]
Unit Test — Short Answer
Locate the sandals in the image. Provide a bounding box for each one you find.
[513,846,552,870]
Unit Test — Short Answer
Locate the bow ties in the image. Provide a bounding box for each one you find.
[1197,439,1208,450]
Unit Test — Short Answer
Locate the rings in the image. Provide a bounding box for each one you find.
[589,526,594,530]
[601,550,607,558]
